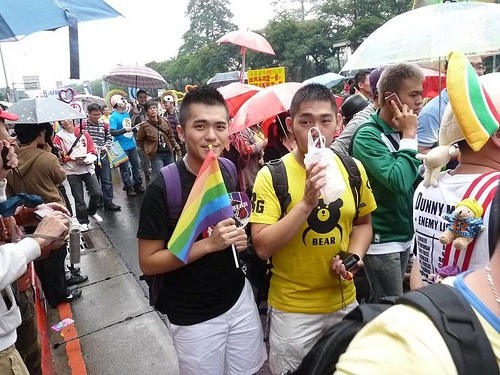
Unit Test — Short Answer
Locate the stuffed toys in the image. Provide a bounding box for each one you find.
[440,198,486,251]
[415,142,459,188]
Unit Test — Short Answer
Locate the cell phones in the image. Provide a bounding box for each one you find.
[33,208,52,220]
[385,93,401,119]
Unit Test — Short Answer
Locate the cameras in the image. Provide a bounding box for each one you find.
[335,251,360,269]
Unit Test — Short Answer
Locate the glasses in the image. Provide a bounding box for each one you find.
[473,66,487,74]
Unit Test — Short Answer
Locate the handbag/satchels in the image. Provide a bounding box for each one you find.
[105,141,129,167]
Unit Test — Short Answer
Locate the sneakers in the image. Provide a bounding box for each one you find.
[52,285,82,307]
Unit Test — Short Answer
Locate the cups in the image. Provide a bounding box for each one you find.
[303,147,346,203]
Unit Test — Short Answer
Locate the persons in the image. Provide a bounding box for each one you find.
[0,54,500,375]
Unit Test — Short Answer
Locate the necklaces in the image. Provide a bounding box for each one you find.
[485,264,500,303]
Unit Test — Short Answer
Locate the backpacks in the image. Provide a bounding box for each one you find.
[289,285,500,375]
[241,149,360,286]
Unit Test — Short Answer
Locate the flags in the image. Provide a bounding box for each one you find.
[167,149,234,266]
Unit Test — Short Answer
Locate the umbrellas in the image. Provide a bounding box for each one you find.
[338,2,500,143]
[0,0,125,101]
[103,62,168,103]
[216,26,278,69]
[72,93,106,107]
[418,66,447,100]
[3,97,87,124]
[228,81,343,151]
[205,71,248,88]
[301,71,344,88]
[216,80,263,118]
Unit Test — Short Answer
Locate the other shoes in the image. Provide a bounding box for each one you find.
[145,177,150,184]
[105,205,120,210]
[134,186,146,194]
[91,213,103,223]
[81,224,88,232]
[65,274,87,284]
[126,189,138,196]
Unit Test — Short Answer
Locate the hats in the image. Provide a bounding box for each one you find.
[161,91,178,107]
[106,90,128,109]
[438,53,500,146]
[0,107,18,121]
